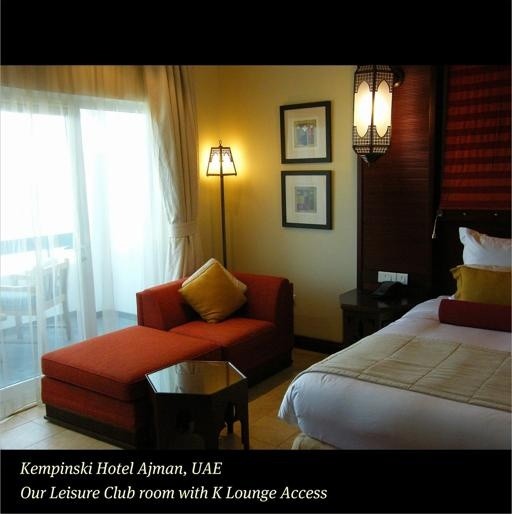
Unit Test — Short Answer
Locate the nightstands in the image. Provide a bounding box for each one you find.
[339,288,406,346]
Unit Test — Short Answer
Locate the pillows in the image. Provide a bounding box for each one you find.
[175,261,248,324]
[451,264,512,307]
[181,258,247,293]
[458,224,512,268]
[437,299,512,334]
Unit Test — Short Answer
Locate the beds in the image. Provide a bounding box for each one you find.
[278,209,512,454]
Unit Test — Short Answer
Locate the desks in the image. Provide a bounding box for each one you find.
[143,359,248,450]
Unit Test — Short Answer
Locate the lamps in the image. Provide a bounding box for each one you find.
[206,140,237,270]
[429,209,444,239]
[353,43,406,167]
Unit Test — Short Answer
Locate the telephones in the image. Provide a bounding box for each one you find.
[369,281,402,302]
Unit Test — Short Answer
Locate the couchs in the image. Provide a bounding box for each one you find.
[41,270,293,450]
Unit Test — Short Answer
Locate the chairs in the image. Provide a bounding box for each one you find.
[0,257,72,361]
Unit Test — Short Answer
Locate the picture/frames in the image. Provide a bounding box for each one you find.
[280,170,332,230]
[279,100,332,163]
